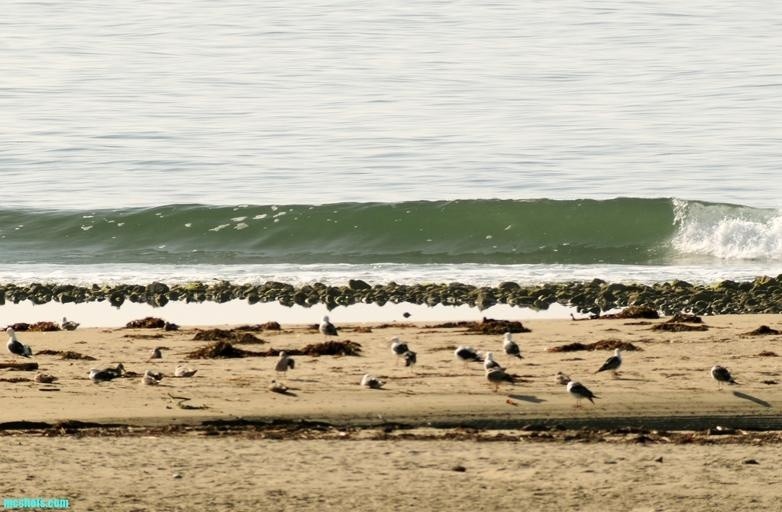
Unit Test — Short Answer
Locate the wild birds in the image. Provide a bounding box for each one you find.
[710,363,740,389]
[4,313,625,408]
